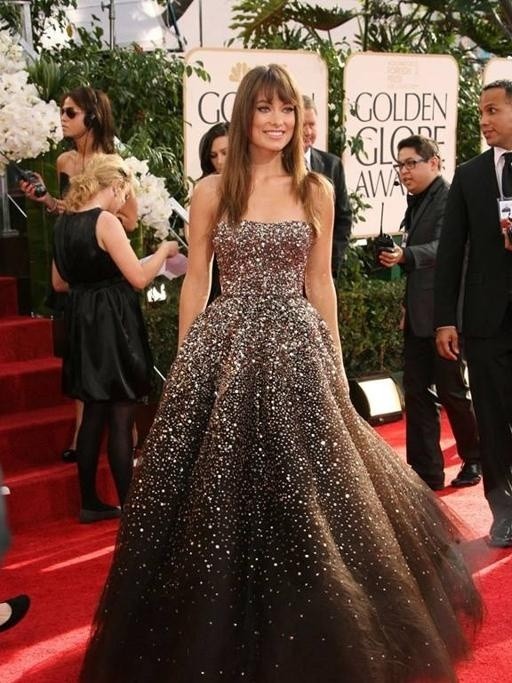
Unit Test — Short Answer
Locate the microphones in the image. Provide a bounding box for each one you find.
[393,138,440,187]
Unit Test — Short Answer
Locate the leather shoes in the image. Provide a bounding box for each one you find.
[489,519,512,548]
[62,447,79,463]
[451,460,481,487]
[77,505,126,524]
[1,595,31,633]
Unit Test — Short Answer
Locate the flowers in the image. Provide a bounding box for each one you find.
[0,20,64,175]
[122,153,173,242]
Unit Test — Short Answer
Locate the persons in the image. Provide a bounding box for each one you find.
[300,94,352,318]
[380,137,485,490]
[52,154,180,522]
[20,87,144,461]
[78,64,485,683]
[194,122,229,311]
[434,79,511,548]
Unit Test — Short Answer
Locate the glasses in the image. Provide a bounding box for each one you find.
[59,105,86,119]
[392,157,431,168]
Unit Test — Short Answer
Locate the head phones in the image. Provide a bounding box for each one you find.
[69,85,99,128]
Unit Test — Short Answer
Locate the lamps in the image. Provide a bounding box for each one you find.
[351,375,403,425]
